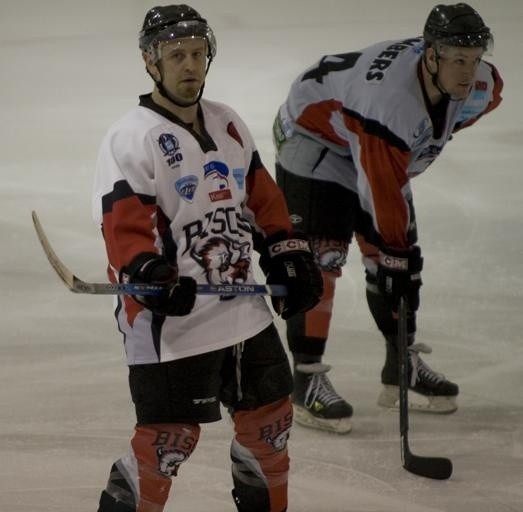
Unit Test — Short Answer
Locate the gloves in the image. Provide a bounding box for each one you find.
[377,246,423,313]
[118,251,196,315]
[259,234,324,320]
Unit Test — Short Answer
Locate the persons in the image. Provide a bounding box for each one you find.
[273,3,504,418]
[93,3,324,512]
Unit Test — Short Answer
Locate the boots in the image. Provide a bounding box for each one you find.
[290,351,353,418]
[382,340,459,396]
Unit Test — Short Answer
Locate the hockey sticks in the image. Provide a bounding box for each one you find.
[32,210,290,296]
[400,295,453,481]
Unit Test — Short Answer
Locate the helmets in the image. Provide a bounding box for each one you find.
[139,4,217,66]
[424,3,494,58]
